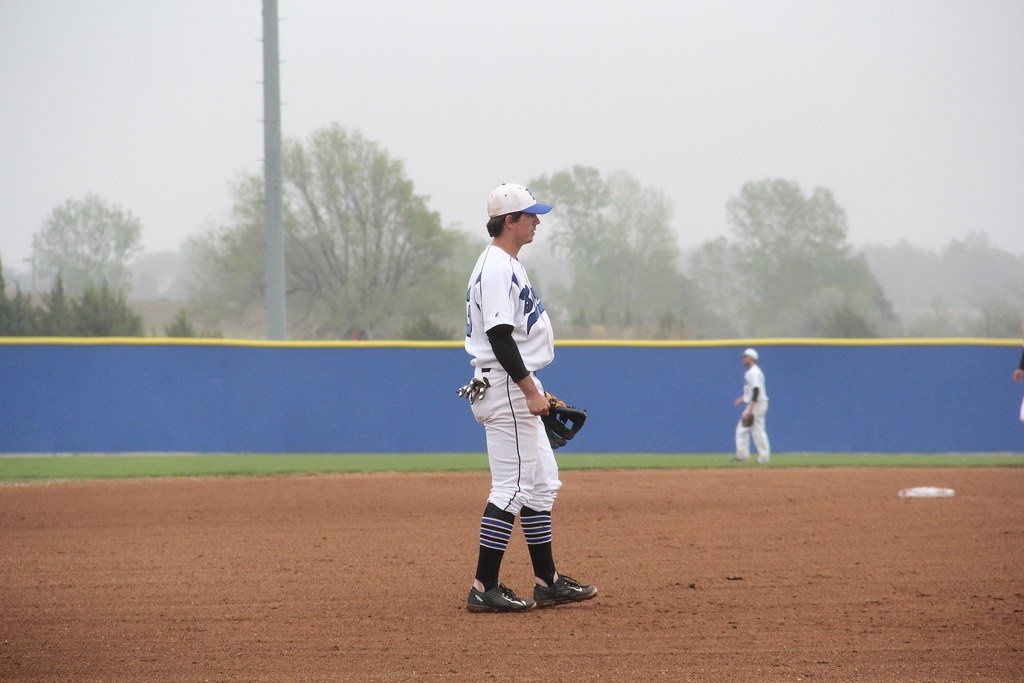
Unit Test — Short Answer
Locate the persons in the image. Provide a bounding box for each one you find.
[1013,348,1024,421]
[464,183,598,616]
[731,348,770,463]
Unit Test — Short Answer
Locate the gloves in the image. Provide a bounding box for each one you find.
[455,375,491,405]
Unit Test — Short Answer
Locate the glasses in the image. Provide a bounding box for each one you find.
[743,354,747,358]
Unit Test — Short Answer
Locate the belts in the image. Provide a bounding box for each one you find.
[481,368,537,377]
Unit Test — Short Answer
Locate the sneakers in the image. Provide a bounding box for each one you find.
[465,580,537,614]
[532,573,599,610]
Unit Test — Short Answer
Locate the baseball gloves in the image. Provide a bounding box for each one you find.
[743,413,754,427]
[541,390,589,449]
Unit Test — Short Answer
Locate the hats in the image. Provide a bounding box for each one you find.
[487,183,554,218]
[744,348,758,362]
